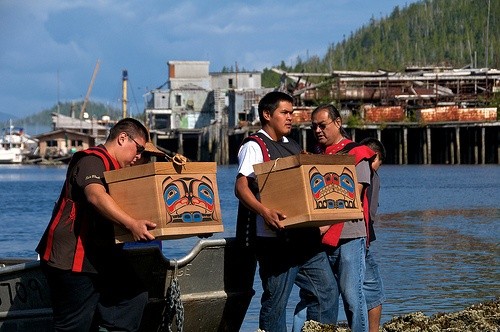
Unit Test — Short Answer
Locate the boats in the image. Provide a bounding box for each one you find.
[0,237,255,332]
[0,118,38,164]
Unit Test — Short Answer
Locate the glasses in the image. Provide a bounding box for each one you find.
[310,117,337,130]
[122,130,145,154]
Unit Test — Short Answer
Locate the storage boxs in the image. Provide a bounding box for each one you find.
[103,162,223,243]
[253,154,364,230]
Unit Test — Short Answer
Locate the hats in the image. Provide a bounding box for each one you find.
[140,126,165,157]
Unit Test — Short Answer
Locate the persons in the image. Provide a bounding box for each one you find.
[35,117,156,332]
[335,137,386,332]
[236,92,339,332]
[292,104,378,332]
